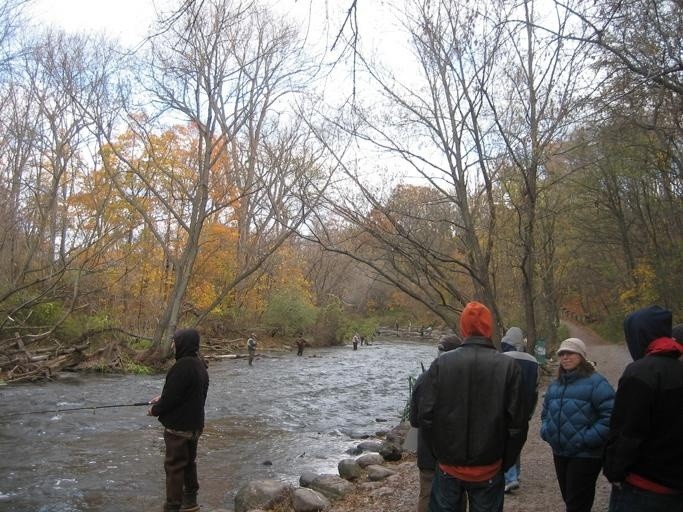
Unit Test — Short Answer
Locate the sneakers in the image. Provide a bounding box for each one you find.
[504,481,520,492]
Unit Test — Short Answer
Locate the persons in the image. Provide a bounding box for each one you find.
[539,337,617,512]
[408,335,467,511]
[352,334,358,351]
[671,324,682,361]
[295,332,306,356]
[414,302,529,511]
[602,303,682,512]
[246,333,256,366]
[497,326,540,494]
[147,329,208,511]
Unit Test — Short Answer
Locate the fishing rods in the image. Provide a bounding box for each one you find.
[0,403,155,416]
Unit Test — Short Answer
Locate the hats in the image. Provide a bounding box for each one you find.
[557,337,586,358]
